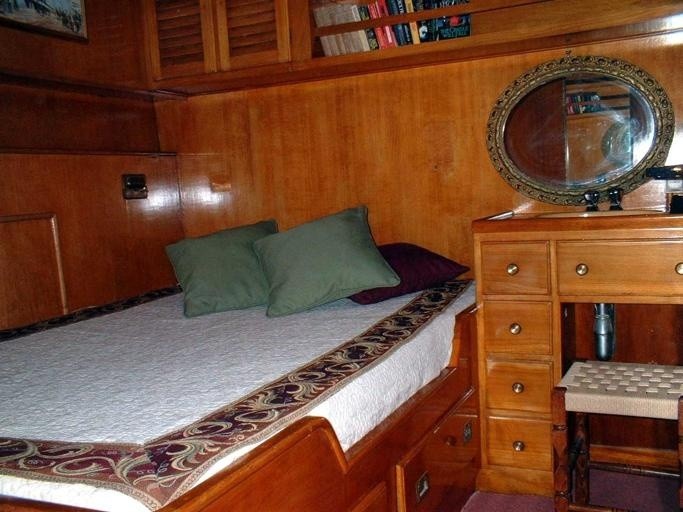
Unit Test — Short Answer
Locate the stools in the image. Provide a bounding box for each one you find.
[551,361,683,510]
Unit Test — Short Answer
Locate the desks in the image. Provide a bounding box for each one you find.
[474,213,683,493]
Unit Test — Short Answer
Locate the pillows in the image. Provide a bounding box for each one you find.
[163,219,277,319]
[347,243,469,305]
[253,205,399,319]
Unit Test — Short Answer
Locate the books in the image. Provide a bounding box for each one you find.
[567,91,600,114]
[310,0,469,56]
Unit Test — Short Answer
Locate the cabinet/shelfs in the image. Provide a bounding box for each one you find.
[306,1,682,78]
[138,1,291,86]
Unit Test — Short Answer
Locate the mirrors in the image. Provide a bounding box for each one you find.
[486,56,675,207]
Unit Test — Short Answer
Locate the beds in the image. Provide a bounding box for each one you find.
[0,279,480,511]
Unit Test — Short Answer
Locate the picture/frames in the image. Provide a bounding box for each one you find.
[0,0,87,42]
